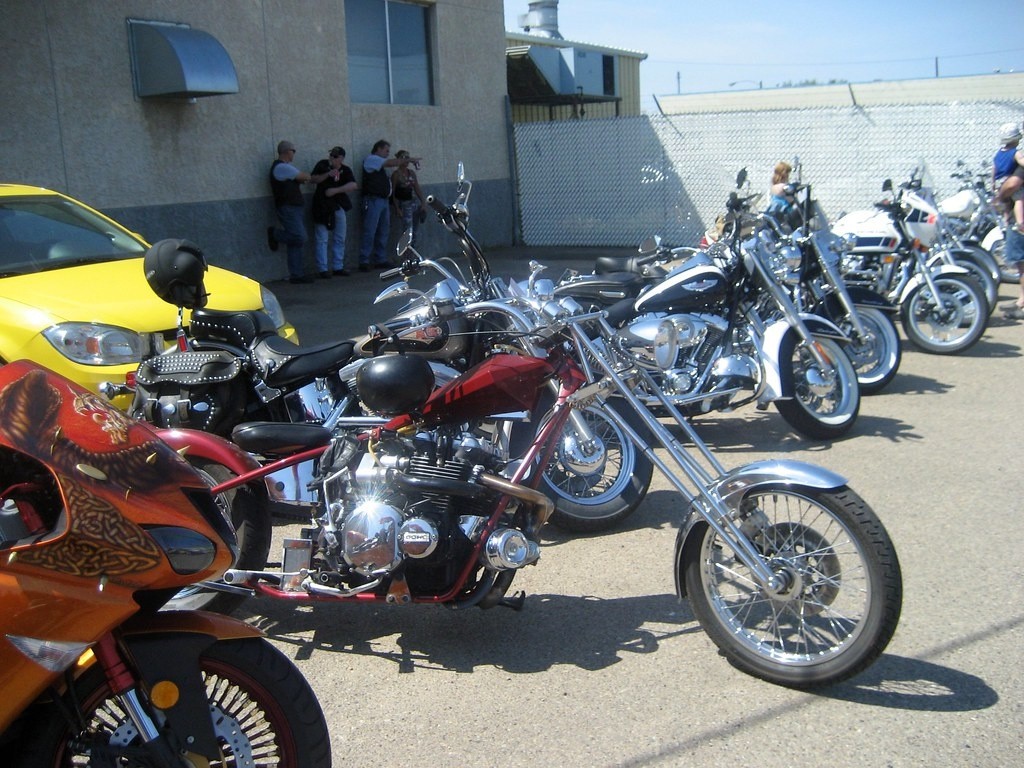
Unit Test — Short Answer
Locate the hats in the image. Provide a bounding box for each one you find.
[329,147,345,158]
[997,123,1022,145]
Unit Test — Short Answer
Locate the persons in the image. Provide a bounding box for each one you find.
[769,162,796,211]
[267,140,359,282]
[991,120,1024,319]
[358,140,427,272]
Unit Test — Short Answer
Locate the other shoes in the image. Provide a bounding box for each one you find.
[359,263,371,272]
[376,262,397,269]
[334,269,351,277]
[291,276,315,285]
[1012,224,1024,234]
[1003,310,1024,321]
[317,271,330,278]
[999,301,1023,311]
[267,226,278,251]
[1002,226,1008,233]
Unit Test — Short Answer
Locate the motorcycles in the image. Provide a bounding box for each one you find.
[0,156,1022,768]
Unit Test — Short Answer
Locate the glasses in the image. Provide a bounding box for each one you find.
[330,153,343,159]
[288,149,296,154]
[400,156,409,159]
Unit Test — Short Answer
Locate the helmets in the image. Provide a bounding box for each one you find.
[356,355,434,415]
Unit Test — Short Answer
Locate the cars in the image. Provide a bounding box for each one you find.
[0,181,302,450]
